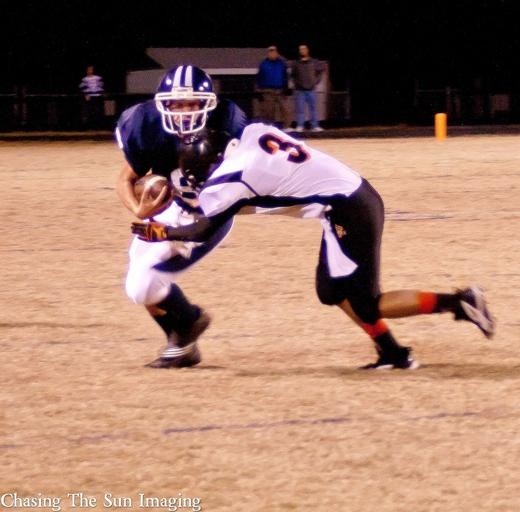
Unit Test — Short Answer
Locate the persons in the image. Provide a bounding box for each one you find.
[254,45,294,132]
[112,64,248,370]
[130,120,496,371]
[289,43,326,132]
[78,63,107,131]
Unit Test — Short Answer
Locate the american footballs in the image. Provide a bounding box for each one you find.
[134,174,173,206]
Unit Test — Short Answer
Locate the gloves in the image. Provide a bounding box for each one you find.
[130,217,169,242]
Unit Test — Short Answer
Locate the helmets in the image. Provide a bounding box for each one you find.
[174,137,220,185]
[153,61,215,136]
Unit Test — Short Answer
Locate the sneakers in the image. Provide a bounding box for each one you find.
[451,283,497,341]
[356,350,421,374]
[141,305,212,370]
[278,125,326,134]
